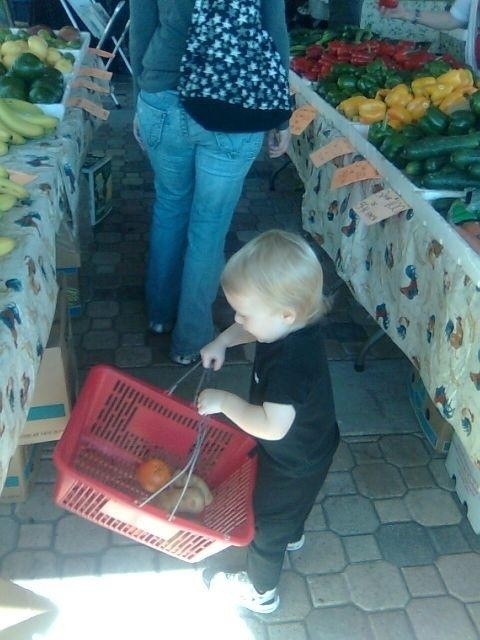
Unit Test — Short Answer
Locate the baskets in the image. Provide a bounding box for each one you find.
[41,361,264,567]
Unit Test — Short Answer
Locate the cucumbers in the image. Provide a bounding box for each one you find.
[400,131,480,191]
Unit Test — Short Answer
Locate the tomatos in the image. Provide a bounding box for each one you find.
[135,459,170,492]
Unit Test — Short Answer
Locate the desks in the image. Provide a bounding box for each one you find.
[0,47,106,498]
[281,58,480,461]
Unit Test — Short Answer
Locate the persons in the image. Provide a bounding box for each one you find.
[371,1,480,83]
[196,225,344,617]
[118,0,296,368]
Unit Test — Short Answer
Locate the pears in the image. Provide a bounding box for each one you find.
[0,34,73,73]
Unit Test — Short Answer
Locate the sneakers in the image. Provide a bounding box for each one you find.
[283,531,307,553]
[149,320,223,368]
[211,570,280,615]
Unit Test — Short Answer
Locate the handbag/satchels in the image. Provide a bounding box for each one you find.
[175,1,294,137]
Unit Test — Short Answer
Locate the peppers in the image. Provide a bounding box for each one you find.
[289,37,480,161]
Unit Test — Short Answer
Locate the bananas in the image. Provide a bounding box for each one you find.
[0,97,58,257]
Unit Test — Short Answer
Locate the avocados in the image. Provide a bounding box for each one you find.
[0,52,64,104]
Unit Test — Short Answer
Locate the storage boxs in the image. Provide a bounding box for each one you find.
[404,360,479,535]
[0,275,79,505]
[56,149,115,316]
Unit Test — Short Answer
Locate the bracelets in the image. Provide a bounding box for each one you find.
[410,8,421,26]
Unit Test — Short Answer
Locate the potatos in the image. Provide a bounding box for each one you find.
[160,486,205,516]
[174,468,213,508]
